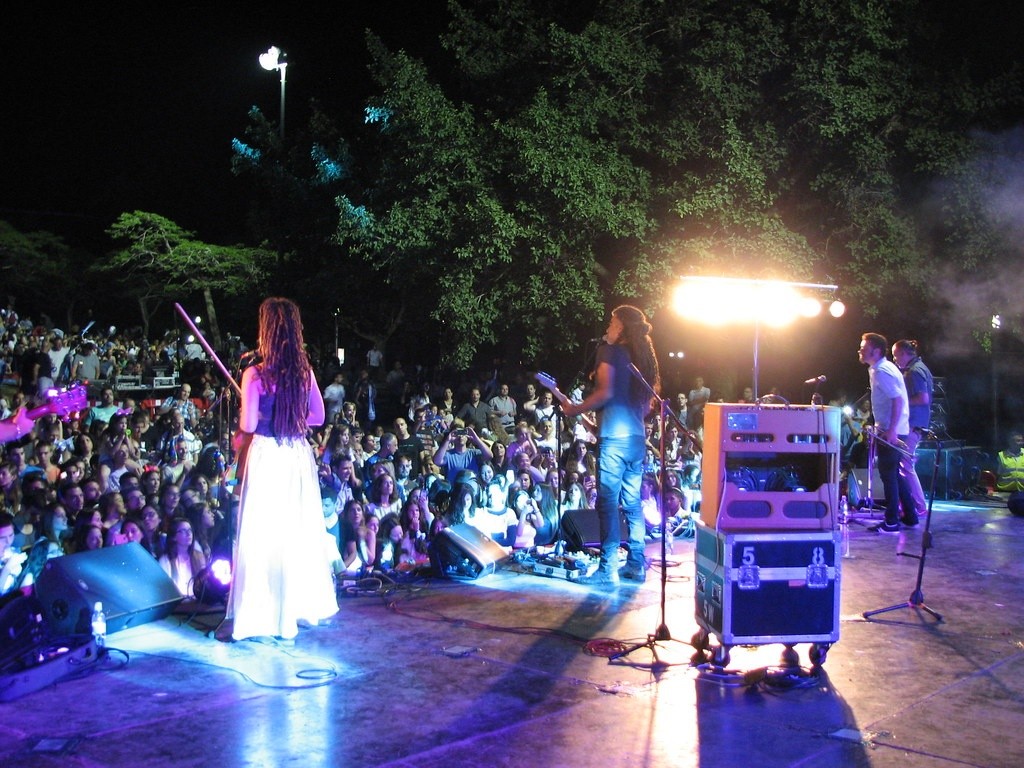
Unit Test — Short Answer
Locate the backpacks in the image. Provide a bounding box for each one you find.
[539,406,555,424]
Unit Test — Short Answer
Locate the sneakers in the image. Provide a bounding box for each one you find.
[866,521,902,534]
[897,520,920,529]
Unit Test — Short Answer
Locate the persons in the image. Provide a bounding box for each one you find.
[995,431,1024,500]
[225,296,339,643]
[857,333,919,535]
[891,339,932,519]
[0,300,873,609]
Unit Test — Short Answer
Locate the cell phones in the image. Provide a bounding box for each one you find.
[457,429,468,435]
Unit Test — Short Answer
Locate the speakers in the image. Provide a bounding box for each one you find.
[700,403,841,533]
[555,508,630,552]
[915,444,981,500]
[34,540,185,637]
[847,468,887,508]
[426,523,510,580]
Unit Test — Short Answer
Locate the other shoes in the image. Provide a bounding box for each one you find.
[618,562,646,582]
[577,569,619,584]
[916,509,928,517]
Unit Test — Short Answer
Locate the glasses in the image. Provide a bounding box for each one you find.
[176,528,192,534]
[126,495,146,501]
[140,512,155,521]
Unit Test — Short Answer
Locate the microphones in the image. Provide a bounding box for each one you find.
[805,376,826,384]
[590,334,609,342]
[242,349,261,359]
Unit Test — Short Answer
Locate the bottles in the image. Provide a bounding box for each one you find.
[840,523,849,558]
[839,496,849,524]
[214,450,225,471]
[665,523,673,555]
[91,601,106,647]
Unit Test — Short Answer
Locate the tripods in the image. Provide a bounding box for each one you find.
[863,428,943,622]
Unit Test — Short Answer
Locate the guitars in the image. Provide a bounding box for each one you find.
[533,370,600,437]
